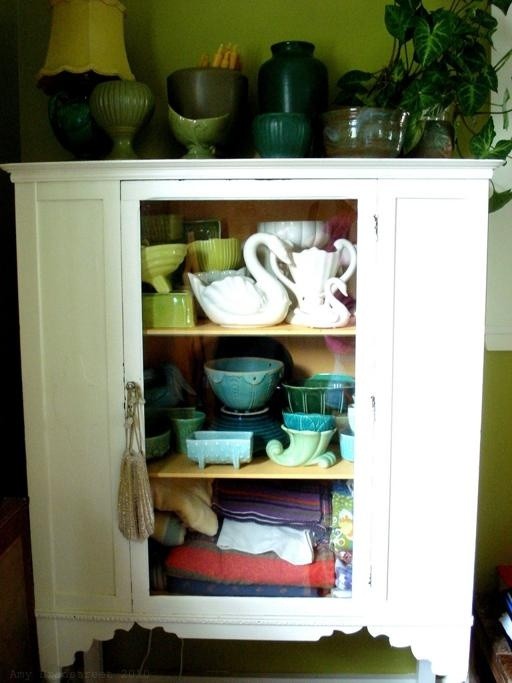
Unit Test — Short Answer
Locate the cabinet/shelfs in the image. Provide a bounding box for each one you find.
[0,156,504,682]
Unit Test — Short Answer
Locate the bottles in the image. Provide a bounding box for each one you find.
[164,41,328,157]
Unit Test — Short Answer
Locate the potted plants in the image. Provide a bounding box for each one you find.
[334,1,512,215]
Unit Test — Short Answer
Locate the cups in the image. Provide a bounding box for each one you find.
[143,407,207,461]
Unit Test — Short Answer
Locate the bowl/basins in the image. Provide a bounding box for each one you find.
[200,357,356,462]
